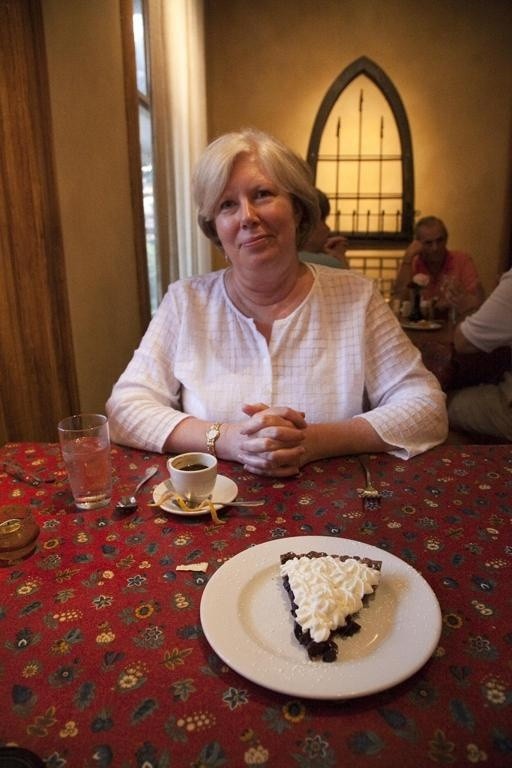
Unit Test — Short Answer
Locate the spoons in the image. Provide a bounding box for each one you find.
[117,465,157,515]
[173,498,269,509]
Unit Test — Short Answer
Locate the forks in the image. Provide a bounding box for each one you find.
[356,453,383,516]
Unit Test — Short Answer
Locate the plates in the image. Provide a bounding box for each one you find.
[400,321,442,330]
[152,474,238,514]
[201,536,441,703]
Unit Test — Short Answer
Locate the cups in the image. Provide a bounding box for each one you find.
[164,452,219,501]
[389,298,457,320]
[1,505,40,562]
[55,411,117,512]
[371,276,383,296]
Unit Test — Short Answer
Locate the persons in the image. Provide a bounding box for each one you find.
[447,268,512,446]
[298,188,350,270]
[105,127,449,476]
[391,216,485,319]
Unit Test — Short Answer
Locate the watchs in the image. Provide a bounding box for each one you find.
[206,422,222,457]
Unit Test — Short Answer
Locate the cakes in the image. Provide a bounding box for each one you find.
[279,549,382,658]
